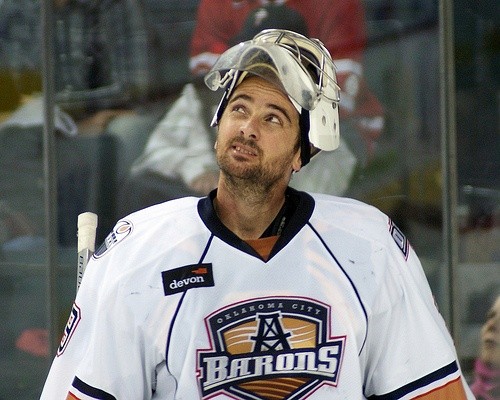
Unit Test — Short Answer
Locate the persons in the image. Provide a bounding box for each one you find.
[39,29,476,400]
[474,293,500,400]
[0,0,387,246]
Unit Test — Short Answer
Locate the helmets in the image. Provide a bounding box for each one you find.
[210,28,341,151]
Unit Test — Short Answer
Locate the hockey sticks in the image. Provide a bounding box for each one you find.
[75,208,104,292]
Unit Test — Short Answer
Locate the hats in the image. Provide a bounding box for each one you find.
[226,6,309,44]
